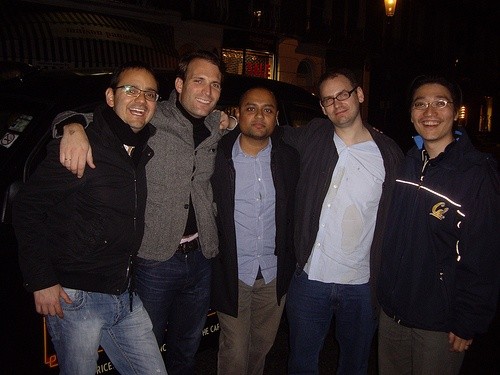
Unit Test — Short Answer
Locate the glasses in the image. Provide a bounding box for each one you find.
[320,86,355,107]
[113,86,160,102]
[412,99,454,110]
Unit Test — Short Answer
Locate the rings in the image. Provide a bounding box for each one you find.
[65,158,71,162]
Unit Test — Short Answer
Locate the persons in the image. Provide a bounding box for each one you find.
[210,67,407,375]
[371,71,500,375]
[50,48,238,375]
[206,78,305,375]
[11,61,168,375]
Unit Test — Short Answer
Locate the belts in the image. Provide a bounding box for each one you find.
[174,236,200,254]
